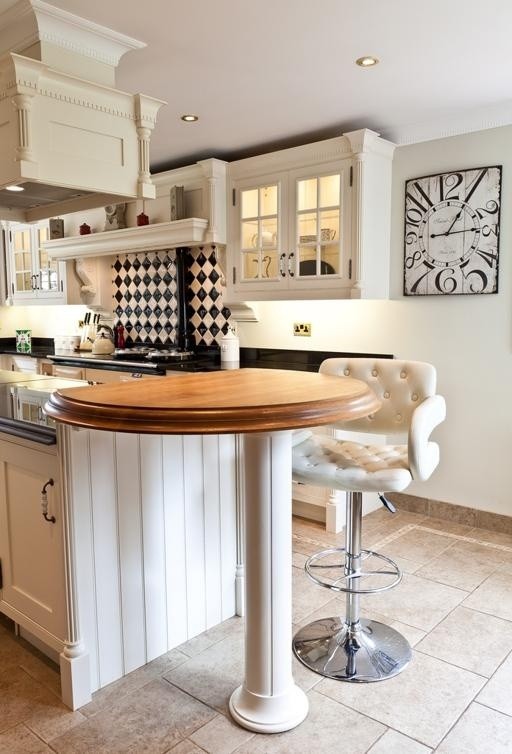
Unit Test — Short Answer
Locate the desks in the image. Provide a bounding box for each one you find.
[47,370,377,735]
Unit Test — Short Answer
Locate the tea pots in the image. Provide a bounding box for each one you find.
[86,324,115,355]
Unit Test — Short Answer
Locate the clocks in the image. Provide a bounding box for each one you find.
[402,165,502,297]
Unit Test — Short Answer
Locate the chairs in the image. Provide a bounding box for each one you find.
[293,358,448,682]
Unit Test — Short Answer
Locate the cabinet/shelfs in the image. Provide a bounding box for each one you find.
[10,355,48,375]
[43,358,85,379]
[7,219,85,306]
[83,369,160,389]
[0,387,68,706]
[226,129,402,301]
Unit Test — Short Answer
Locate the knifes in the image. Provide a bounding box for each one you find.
[83,312,101,326]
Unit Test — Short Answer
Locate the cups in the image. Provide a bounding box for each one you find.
[70,335,81,352]
